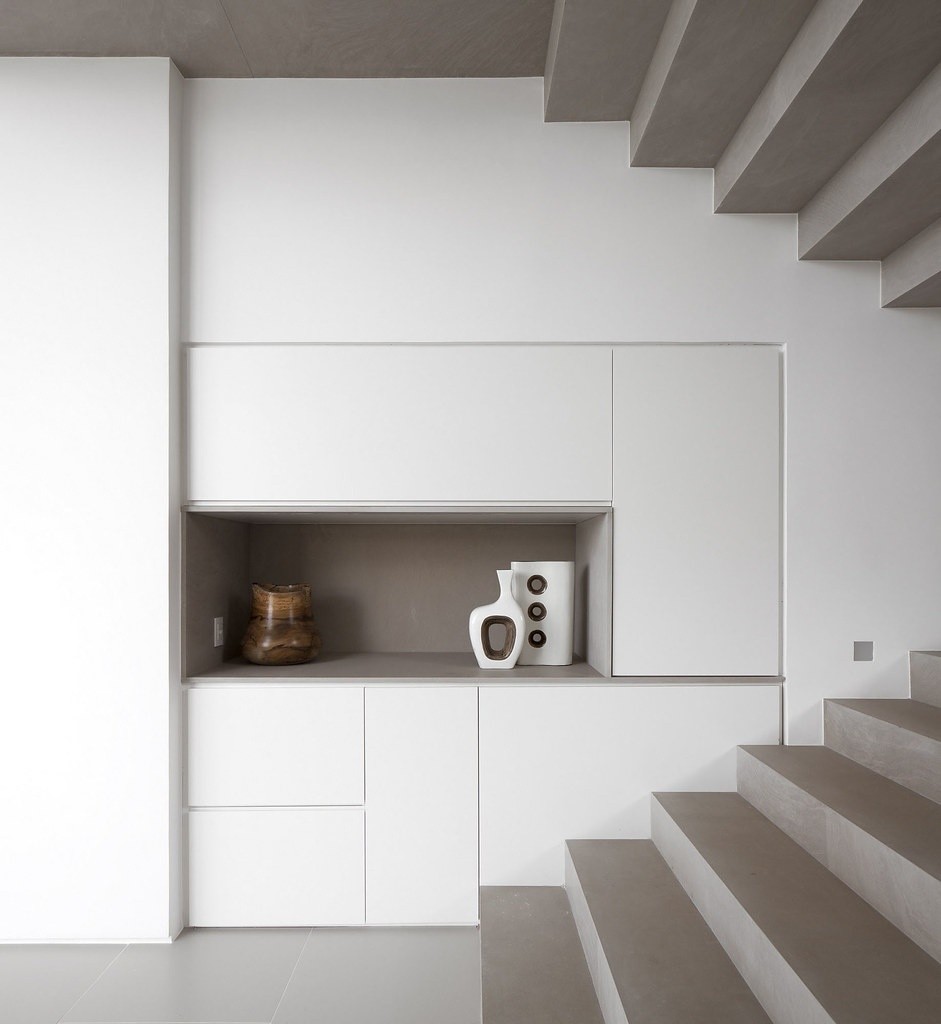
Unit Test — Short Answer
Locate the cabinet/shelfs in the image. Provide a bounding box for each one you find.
[181,344,781,928]
